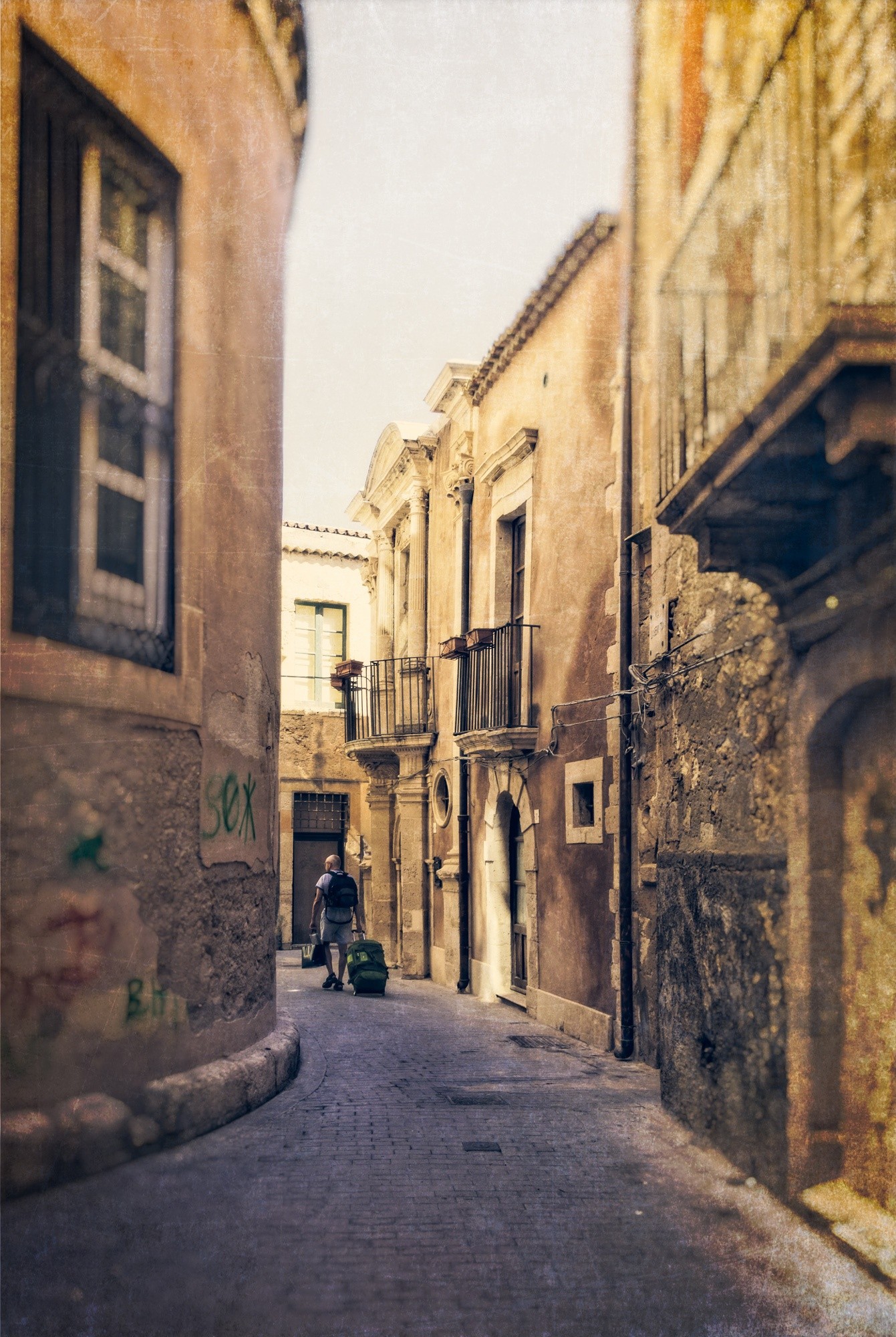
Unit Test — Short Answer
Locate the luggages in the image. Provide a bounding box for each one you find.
[345,930,389,996]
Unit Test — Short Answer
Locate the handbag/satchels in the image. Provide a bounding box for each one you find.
[302,928,327,969]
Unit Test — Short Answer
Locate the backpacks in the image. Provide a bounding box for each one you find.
[323,871,359,924]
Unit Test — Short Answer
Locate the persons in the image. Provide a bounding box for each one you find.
[309,855,362,990]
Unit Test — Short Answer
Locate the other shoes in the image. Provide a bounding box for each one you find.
[322,972,337,988]
[333,980,344,990]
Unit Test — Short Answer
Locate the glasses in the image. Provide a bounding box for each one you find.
[324,862,330,864]
